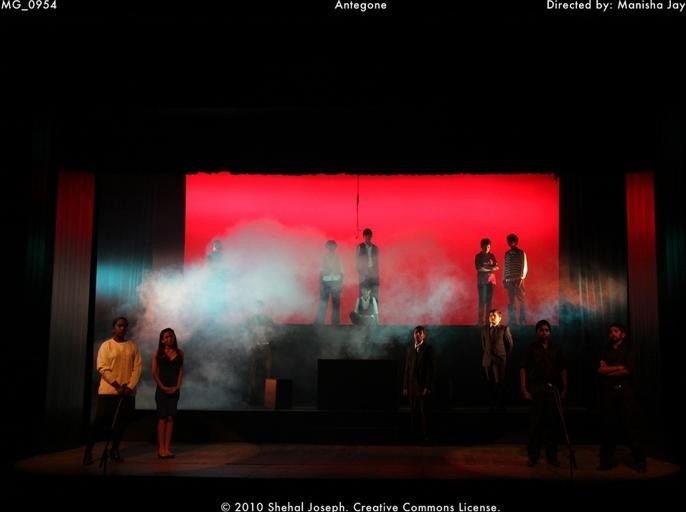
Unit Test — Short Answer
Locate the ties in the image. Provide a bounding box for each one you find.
[492,326,497,336]
[413,347,418,365]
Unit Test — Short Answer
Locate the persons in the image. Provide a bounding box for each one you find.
[82,315,142,465]
[354,228,380,302]
[596,322,648,473]
[473,238,499,326]
[402,326,435,447]
[503,233,528,326]
[481,308,513,406]
[206,239,226,272]
[244,300,279,407]
[520,319,567,469]
[349,280,380,327]
[150,327,184,459]
[316,241,344,326]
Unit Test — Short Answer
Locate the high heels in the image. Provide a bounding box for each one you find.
[157,448,176,460]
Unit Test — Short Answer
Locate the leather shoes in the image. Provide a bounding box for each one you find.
[526,452,561,467]
[593,461,647,472]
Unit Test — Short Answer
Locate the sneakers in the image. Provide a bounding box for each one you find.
[507,320,525,326]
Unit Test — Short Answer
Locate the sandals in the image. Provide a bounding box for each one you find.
[114,447,125,462]
[83,449,92,465]
